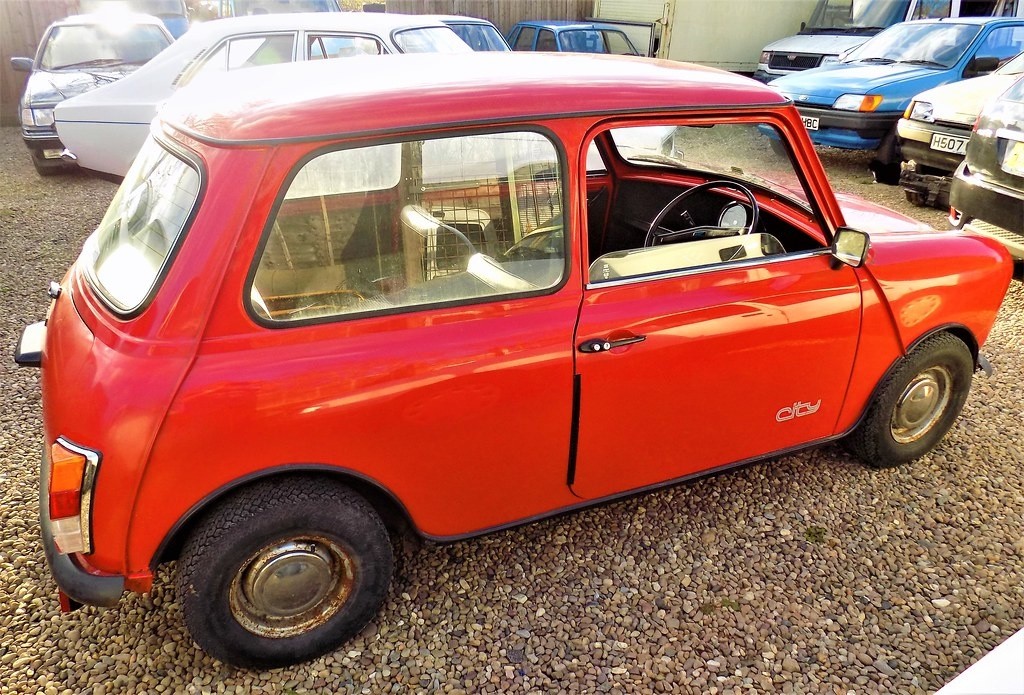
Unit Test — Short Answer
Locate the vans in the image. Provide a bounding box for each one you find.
[753,0,1023,86]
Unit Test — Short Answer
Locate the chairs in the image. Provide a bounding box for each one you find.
[403,203,473,285]
[468,252,539,291]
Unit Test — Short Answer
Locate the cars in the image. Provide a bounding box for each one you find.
[506,20,646,58]
[10,8,178,178]
[51,12,686,199]
[13,50,1015,670]
[218,0,356,62]
[758,16,1024,169]
[895,51,1023,211]
[947,74,1024,261]
[433,14,513,53]
